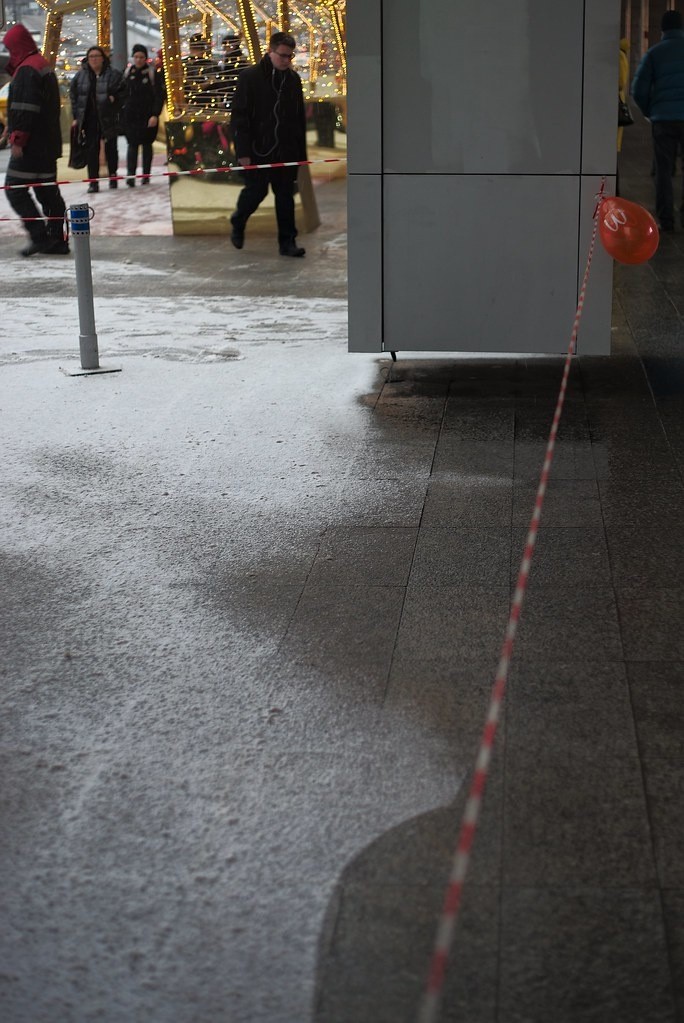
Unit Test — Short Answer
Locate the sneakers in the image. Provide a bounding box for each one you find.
[230,214,244,249]
[279,243,305,256]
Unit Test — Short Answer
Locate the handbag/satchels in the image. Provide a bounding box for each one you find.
[67,127,88,170]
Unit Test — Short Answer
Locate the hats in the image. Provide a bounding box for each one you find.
[132,43,148,59]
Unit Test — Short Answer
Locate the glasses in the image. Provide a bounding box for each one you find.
[273,50,296,60]
[88,54,103,58]
[132,55,146,60]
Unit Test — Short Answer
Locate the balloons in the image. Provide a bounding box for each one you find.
[598,197,659,265]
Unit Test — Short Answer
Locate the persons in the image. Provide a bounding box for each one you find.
[182,33,221,107]
[124,44,168,187]
[69,46,126,189]
[615,10,684,237]
[226,35,250,103]
[3,25,70,256]
[231,33,306,257]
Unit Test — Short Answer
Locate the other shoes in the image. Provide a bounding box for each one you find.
[127,181,134,187]
[142,178,150,184]
[87,183,99,193]
[108,183,116,188]
[21,241,70,256]
